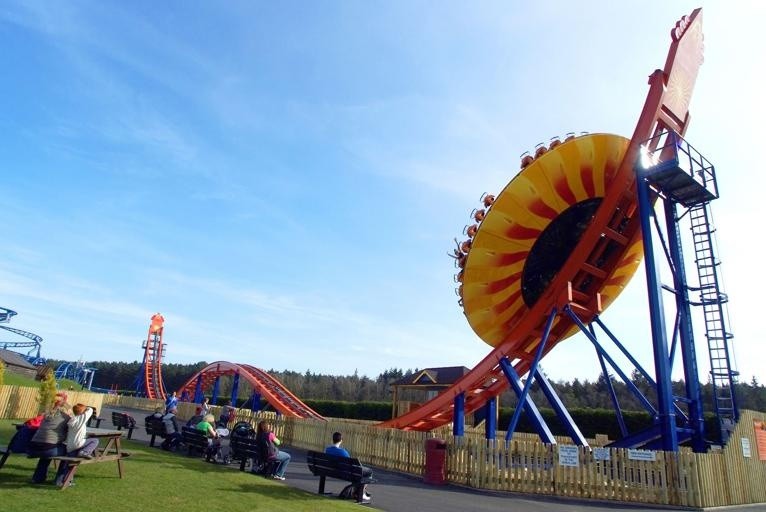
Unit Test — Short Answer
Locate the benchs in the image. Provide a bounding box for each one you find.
[83,406,379,506]
[0,444,136,490]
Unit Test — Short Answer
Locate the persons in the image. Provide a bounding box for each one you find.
[26,400,72,486]
[180,389,191,402]
[165,391,178,415]
[325,432,374,500]
[66,403,99,467]
[447,236,464,260]
[153,398,291,481]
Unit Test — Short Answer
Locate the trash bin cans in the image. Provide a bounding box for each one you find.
[424,438,447,485]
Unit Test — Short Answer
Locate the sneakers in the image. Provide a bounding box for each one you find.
[30,478,53,483]
[271,473,285,481]
[77,450,93,459]
[55,481,75,487]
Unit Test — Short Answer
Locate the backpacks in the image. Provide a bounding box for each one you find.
[338,484,372,500]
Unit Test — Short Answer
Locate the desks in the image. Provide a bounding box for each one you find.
[10,421,125,452]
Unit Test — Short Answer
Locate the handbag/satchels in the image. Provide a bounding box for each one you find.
[228,414,235,422]
[9,426,37,453]
[195,406,202,416]
